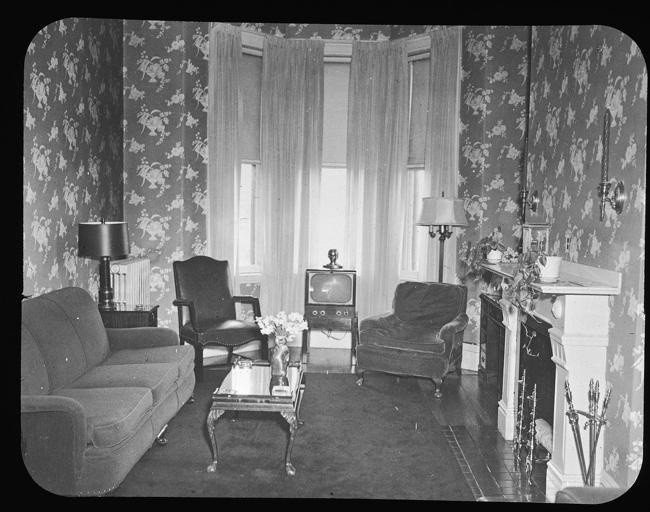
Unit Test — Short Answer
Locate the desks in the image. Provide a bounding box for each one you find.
[295,313,358,371]
[94,297,159,328]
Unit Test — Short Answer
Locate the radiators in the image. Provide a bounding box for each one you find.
[105,256,155,301]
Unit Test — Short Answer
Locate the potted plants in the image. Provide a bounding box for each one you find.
[470,234,507,270]
[498,242,561,320]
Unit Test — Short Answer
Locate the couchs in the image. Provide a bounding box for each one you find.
[20,283,202,497]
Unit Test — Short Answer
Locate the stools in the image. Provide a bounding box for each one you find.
[547,482,630,505]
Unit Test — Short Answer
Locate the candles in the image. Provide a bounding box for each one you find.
[522,135,528,189]
[601,107,611,182]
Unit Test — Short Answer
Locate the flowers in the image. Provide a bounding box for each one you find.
[253,306,311,346]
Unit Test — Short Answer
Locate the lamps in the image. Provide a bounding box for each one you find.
[411,189,473,282]
[75,217,137,313]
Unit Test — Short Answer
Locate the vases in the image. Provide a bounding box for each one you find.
[269,340,291,383]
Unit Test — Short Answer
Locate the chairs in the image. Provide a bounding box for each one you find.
[355,280,468,397]
[172,255,268,379]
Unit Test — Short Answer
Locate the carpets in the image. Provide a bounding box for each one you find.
[103,368,478,502]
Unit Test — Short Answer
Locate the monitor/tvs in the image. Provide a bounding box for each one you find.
[306,271,354,305]
